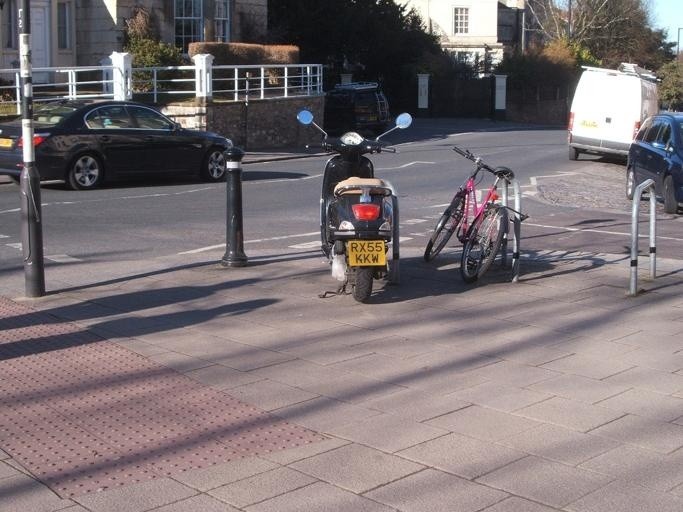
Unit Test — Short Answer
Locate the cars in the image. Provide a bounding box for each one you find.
[625,110,682,215]
[0,99,233,191]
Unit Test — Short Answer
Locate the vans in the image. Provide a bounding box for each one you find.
[566,62,662,161]
[324,81,391,122]
[324,92,369,138]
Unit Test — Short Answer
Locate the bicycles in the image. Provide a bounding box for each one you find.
[424,146,514,285]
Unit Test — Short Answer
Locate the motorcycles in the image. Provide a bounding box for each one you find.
[296,109,413,302]
